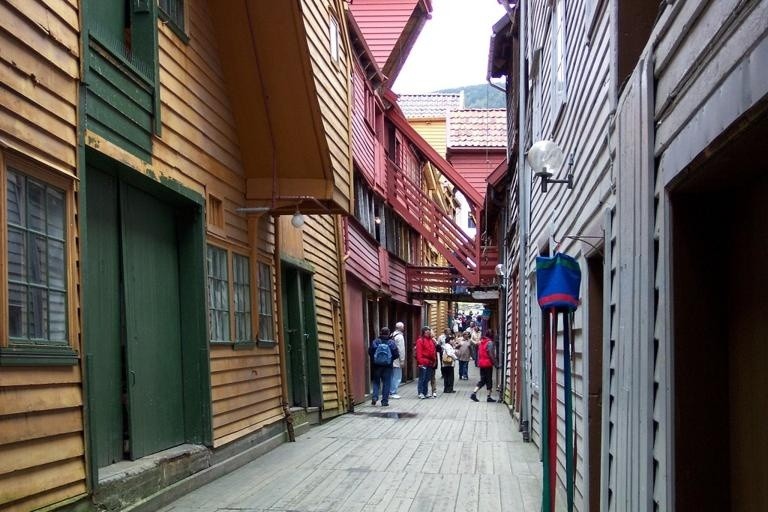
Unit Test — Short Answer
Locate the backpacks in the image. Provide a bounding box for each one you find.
[388,332,399,360]
[372,339,392,365]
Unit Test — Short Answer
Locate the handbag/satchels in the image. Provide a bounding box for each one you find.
[443,353,452,364]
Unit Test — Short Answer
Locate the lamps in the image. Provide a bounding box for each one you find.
[292,200,304,228]
[527,140,574,192]
[495,263,511,285]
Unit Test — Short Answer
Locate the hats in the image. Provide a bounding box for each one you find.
[422,326,430,336]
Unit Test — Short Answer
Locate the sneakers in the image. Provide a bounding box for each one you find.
[487,398,496,402]
[444,389,456,393]
[389,394,400,399]
[372,400,376,405]
[470,393,479,402]
[418,392,437,399]
[460,374,468,380]
[382,403,389,406]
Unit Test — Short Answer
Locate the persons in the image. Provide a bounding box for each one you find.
[416,311,499,402]
[368,327,399,406]
[388,322,405,399]
